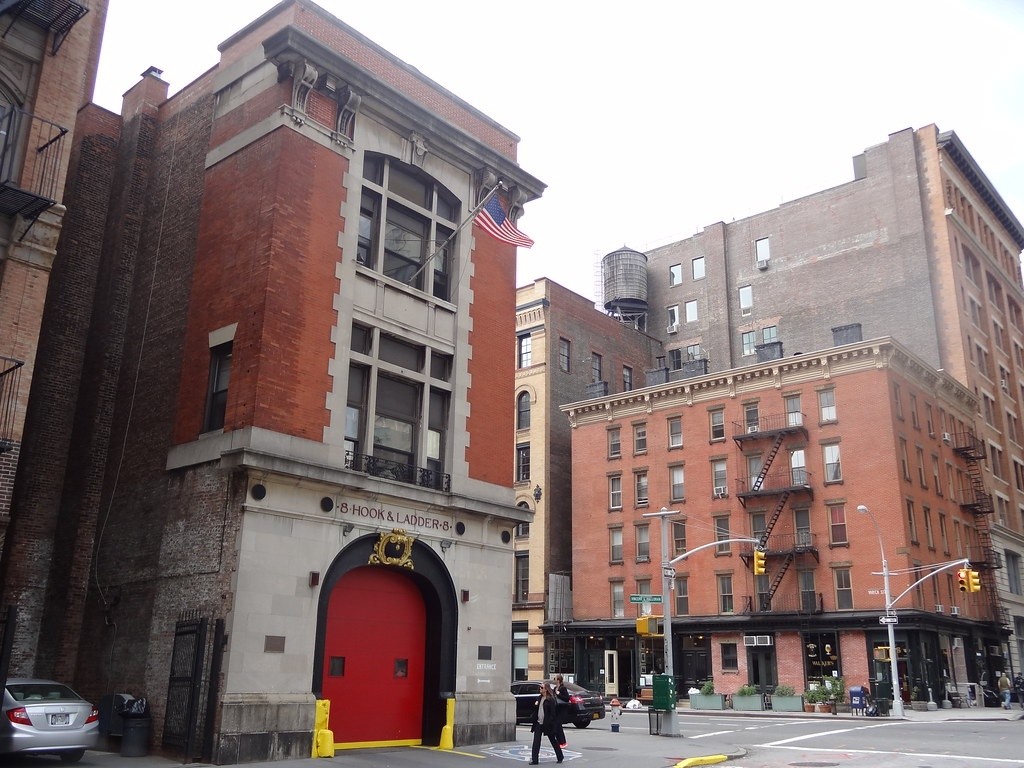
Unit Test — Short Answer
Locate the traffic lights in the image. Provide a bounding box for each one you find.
[872,647,887,661]
[968,568,983,593]
[956,568,970,593]
[635,618,658,635]
[753,547,768,576]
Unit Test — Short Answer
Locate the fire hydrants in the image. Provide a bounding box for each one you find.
[609,698,623,732]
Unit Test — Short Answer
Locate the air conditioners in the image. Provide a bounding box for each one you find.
[950,606,960,615]
[667,325,677,333]
[744,636,756,646]
[757,635,773,646]
[989,646,1002,657]
[749,426,758,432]
[953,638,962,647]
[942,433,951,441]
[757,259,769,269]
[1001,380,1007,388]
[935,605,944,612]
[714,486,725,495]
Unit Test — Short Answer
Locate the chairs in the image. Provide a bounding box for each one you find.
[47,692,61,698]
[14,693,25,700]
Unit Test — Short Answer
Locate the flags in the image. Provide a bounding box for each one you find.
[472,193,534,249]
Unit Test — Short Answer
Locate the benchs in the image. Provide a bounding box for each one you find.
[636,689,653,704]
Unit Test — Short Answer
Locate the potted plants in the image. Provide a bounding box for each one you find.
[815,685,832,713]
[733,685,764,711]
[802,688,816,712]
[689,680,725,710]
[770,685,803,711]
[910,687,928,710]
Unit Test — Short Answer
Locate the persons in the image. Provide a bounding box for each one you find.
[529,682,564,765]
[552,675,569,749]
[1014,672,1024,710]
[998,672,1012,709]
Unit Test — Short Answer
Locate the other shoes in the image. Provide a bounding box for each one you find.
[1009,704,1012,709]
[529,761,538,765]
[557,756,564,763]
[1001,701,1006,708]
[559,742,567,749]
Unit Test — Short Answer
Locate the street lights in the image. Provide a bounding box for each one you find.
[856,504,908,719]
[658,538,752,742]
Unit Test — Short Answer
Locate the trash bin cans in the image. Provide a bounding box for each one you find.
[120,718,150,757]
[648,706,664,735]
[877,698,890,717]
[850,686,868,716]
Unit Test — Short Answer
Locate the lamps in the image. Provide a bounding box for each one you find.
[461,589,470,601]
[310,571,320,585]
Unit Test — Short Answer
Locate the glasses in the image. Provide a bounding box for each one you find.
[554,678,555,680]
[540,686,543,689]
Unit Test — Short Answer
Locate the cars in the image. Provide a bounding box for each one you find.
[0,678,101,762]
[511,680,606,729]
